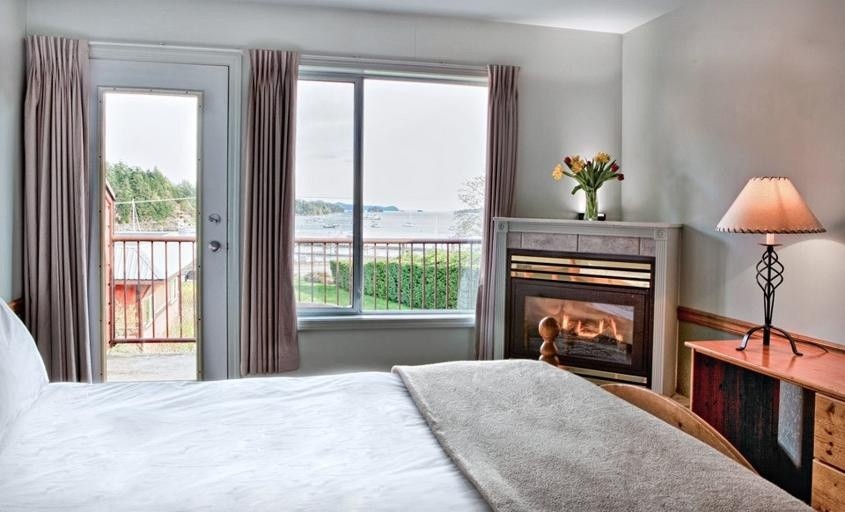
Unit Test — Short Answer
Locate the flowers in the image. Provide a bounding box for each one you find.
[552,152,624,219]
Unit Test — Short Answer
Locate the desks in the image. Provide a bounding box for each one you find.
[685,340,845,512]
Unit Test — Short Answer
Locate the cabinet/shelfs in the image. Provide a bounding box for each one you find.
[491,216,685,398]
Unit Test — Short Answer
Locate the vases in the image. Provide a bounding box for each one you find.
[584,192,598,222]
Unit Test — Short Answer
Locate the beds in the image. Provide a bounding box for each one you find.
[0,303,818,510]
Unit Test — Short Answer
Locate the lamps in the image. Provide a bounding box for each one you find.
[715,176,828,357]
